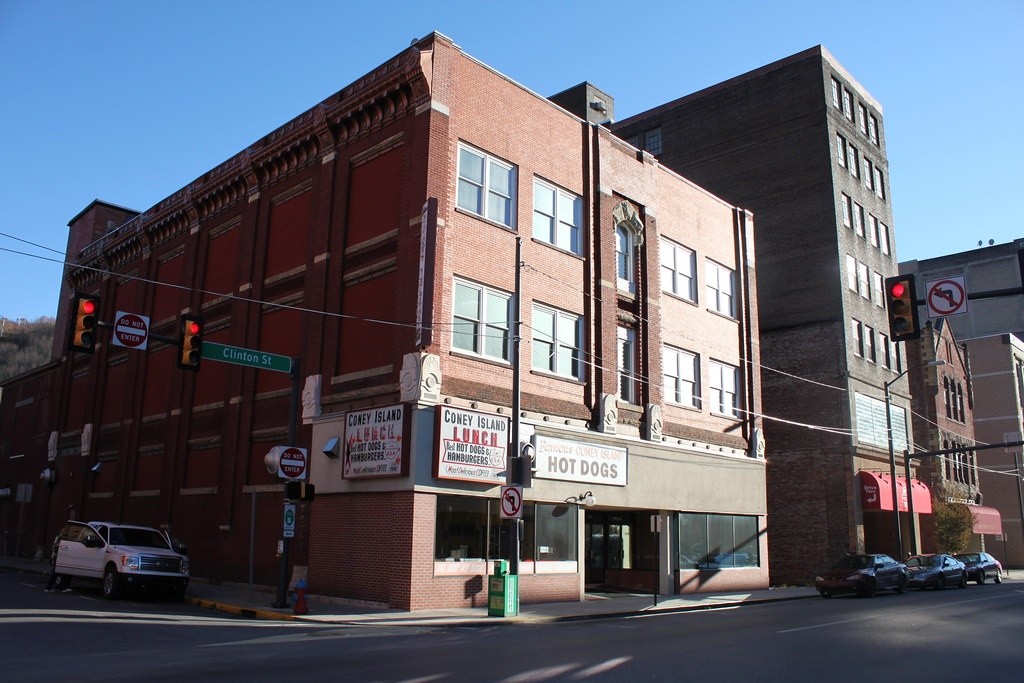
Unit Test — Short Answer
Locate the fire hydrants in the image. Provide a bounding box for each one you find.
[291,577,310,615]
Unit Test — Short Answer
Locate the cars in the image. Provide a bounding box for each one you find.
[952,552,1003,586]
[899,554,969,591]
[815,553,908,599]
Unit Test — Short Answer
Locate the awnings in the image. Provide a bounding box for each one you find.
[950,503,1003,536]
[860,472,932,514]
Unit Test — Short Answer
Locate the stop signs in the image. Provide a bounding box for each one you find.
[115,313,148,348]
[280,448,306,478]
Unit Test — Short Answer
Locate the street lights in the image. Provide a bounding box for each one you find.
[883,360,948,560]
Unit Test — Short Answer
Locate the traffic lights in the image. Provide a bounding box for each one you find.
[177,314,203,372]
[885,272,921,341]
[63,289,101,353]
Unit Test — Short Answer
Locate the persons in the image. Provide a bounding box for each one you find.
[44,527,81,593]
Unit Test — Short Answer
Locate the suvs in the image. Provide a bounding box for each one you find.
[53,520,190,603]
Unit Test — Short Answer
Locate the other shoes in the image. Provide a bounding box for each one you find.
[44,589,55,592]
[61,589,72,593]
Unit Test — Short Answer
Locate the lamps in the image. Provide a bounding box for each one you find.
[579,490,596,506]
